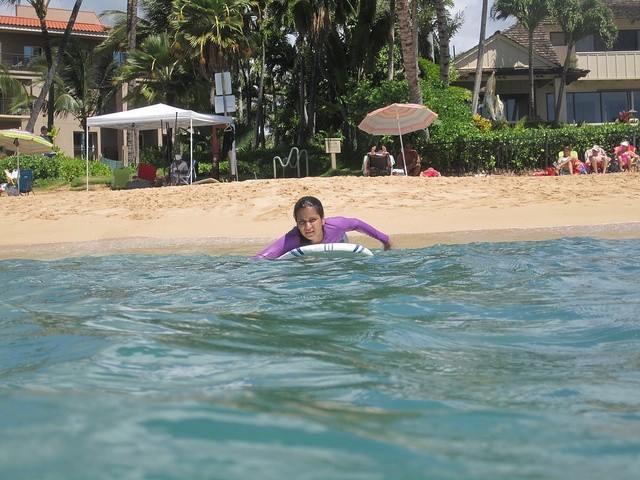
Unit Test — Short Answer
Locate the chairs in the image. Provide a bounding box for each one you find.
[16,169,35,195]
[172,159,196,185]
[559,151,578,174]
[614,145,636,172]
[585,148,606,173]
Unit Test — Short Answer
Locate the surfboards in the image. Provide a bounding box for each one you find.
[276,242,374,260]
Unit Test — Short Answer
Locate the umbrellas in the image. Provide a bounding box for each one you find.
[356,101,440,176]
[0,128,54,196]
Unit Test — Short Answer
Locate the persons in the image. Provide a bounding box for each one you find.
[38,125,50,143]
[361,144,377,176]
[397,141,423,177]
[587,144,608,174]
[251,195,394,261]
[380,146,395,174]
[616,141,640,173]
[555,145,579,175]
[169,153,188,178]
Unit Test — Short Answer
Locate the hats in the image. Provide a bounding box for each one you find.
[592,145,599,152]
[620,141,630,146]
[174,154,183,161]
